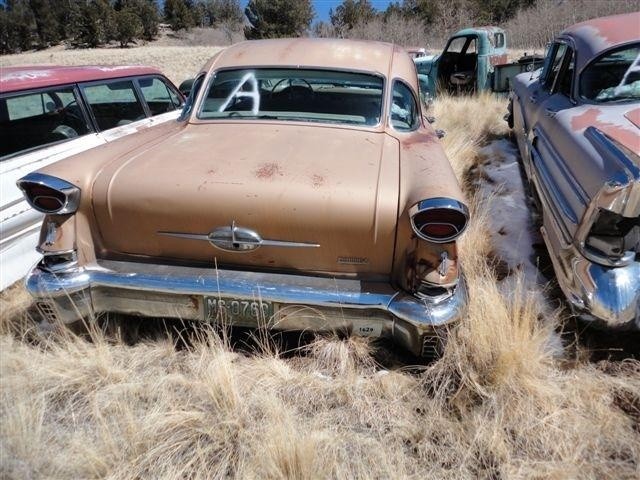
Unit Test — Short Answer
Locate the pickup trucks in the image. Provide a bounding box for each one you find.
[414,24,628,101]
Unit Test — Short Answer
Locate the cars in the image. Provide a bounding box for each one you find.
[15,28,471,360]
[506,14,640,346]
[1,64,186,296]
